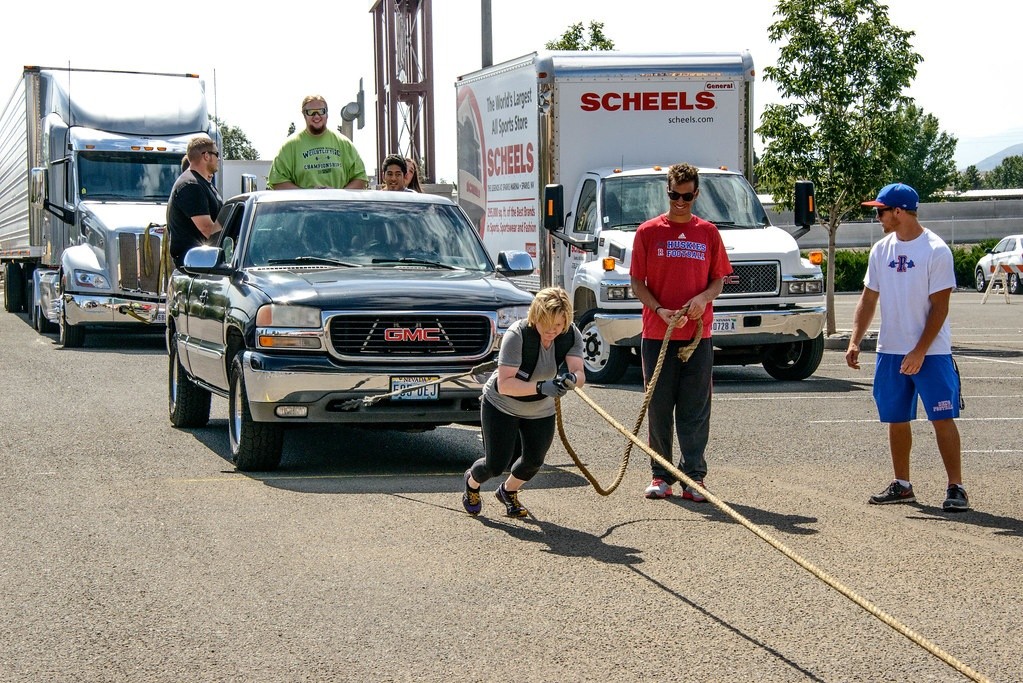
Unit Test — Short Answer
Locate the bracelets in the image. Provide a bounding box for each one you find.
[655,307,663,314]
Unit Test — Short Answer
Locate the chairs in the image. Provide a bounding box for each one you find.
[605,192,629,226]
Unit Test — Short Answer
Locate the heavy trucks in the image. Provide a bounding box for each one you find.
[0,64,276,348]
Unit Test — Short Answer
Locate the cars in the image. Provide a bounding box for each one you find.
[974,235,1023,294]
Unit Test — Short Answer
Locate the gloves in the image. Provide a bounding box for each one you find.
[536,378,567,397]
[560,373,577,389]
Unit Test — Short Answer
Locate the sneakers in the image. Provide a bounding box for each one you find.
[495,483,528,516]
[644,478,673,498]
[462,469,481,514]
[868,480,916,504]
[943,484,970,509]
[683,481,706,502]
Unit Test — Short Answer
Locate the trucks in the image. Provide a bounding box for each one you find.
[453,50,828,384]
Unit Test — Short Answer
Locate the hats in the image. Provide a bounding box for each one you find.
[861,182,919,210]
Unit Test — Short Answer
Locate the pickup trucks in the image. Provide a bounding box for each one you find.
[166,189,536,472]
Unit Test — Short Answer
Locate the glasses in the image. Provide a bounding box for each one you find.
[202,152,219,158]
[667,185,698,201]
[305,108,327,116]
[877,207,892,217]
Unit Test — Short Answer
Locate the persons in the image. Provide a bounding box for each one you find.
[381,154,422,193]
[462,288,589,517]
[166,134,223,264]
[845,183,972,509]
[267,96,369,190]
[628,164,733,501]
